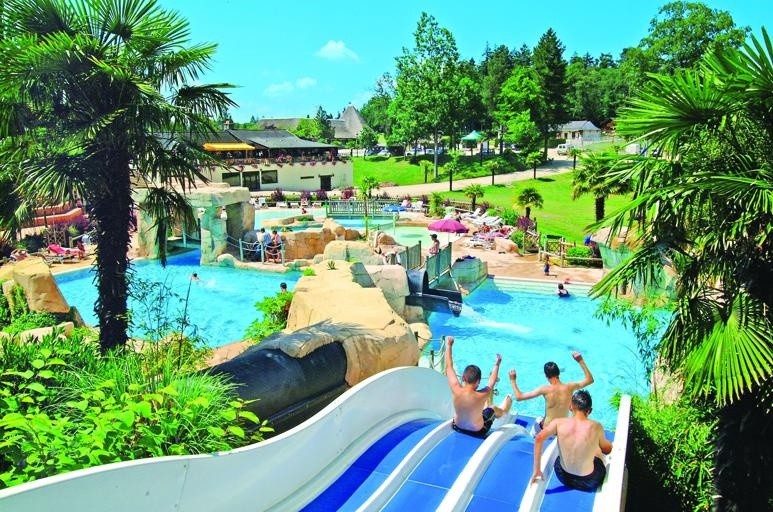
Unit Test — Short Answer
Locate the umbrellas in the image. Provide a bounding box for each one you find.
[427,218,468,246]
[382,205,404,235]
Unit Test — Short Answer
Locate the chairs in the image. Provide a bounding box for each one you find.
[399,199,503,229]
[48,244,82,264]
[249,196,321,209]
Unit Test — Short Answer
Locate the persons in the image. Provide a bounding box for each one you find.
[454,217,461,237]
[191,273,198,279]
[427,233,439,259]
[557,284,570,297]
[43,248,71,258]
[588,241,602,267]
[543,255,549,276]
[532,391,614,492]
[270,230,285,263]
[214,147,336,163]
[444,337,513,439]
[478,222,486,234]
[258,227,276,263]
[508,351,594,436]
[279,281,291,320]
[9,248,29,262]
[301,207,307,214]
[399,192,430,214]
[75,240,85,259]
